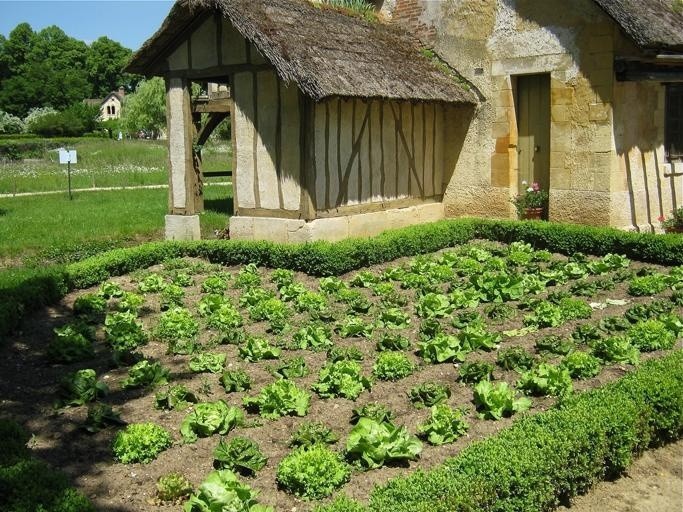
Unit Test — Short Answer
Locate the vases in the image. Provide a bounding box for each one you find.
[665,227,682,233]
[523,208,543,220]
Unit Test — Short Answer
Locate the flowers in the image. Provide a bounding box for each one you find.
[521,179,547,207]
[656,206,682,227]
